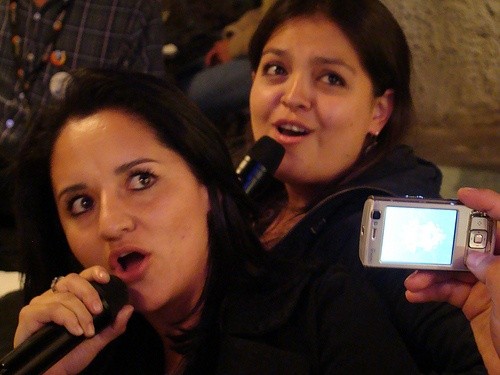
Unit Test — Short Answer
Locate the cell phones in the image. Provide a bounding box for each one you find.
[360,194,497,271]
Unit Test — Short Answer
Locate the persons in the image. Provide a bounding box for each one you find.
[169,0,284,69]
[403,186,500,375]
[236,0,499,375]
[0,70,381,374]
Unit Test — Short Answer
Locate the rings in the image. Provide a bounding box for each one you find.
[51,275,65,291]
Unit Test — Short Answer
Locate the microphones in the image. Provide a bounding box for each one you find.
[235,134,287,197]
[0,274,128,375]
